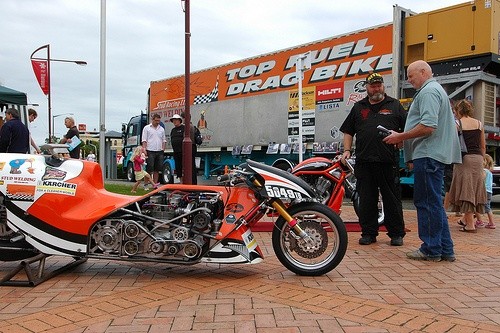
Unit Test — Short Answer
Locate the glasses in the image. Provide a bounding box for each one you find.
[32,115,36,119]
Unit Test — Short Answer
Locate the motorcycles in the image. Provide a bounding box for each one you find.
[0,127,385,276]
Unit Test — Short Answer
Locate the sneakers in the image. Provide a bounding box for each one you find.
[391,235,403,245]
[443,255,455,262]
[406,249,441,261]
[358,234,376,245]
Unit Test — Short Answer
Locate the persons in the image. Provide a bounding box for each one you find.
[443,98,496,232]
[21,108,41,154]
[154,110,167,184]
[130,146,160,193]
[142,113,167,191]
[170,111,202,185]
[382,60,462,262]
[59,116,80,158]
[339,72,408,247]
[0,108,29,153]
[87,150,95,162]
[0,116,4,129]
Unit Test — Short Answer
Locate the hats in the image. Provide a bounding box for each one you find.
[169,114,183,123]
[366,73,384,84]
[200,111,205,117]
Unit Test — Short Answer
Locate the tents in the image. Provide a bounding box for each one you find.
[0,85,31,154]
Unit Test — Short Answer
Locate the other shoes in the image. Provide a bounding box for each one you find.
[131,189,137,193]
[475,221,484,227]
[485,223,495,229]
[153,182,160,188]
[144,184,149,191]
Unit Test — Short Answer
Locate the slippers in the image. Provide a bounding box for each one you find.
[457,220,466,226]
[459,227,477,233]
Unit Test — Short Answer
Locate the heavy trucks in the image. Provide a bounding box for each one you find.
[120,0,500,196]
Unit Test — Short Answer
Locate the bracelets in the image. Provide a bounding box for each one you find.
[345,149,350,151]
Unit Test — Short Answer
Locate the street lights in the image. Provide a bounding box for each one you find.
[53,112,76,138]
[30,43,88,143]
